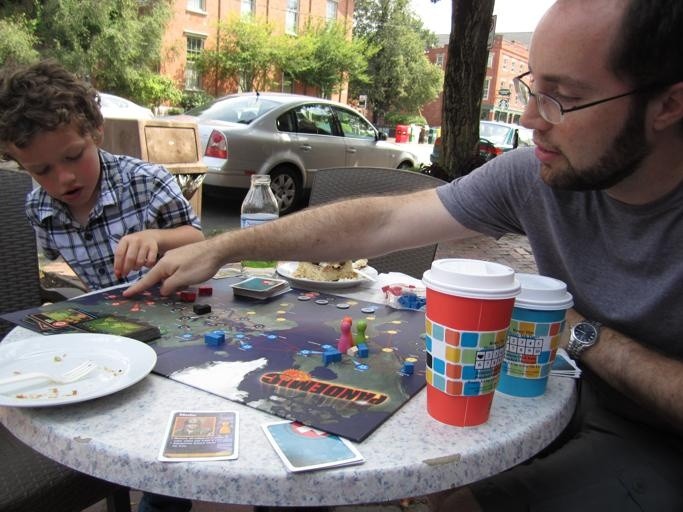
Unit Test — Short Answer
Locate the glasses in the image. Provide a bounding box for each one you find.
[513,72,632,125]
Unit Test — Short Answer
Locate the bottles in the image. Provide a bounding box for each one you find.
[238,171,280,278]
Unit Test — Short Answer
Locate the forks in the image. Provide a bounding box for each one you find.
[1,357,95,392]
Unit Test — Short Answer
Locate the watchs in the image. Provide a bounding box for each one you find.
[565,318,603,363]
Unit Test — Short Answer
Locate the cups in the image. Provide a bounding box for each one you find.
[421,257,574,425]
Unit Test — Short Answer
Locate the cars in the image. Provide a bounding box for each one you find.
[429,119,533,169]
[169,93,417,219]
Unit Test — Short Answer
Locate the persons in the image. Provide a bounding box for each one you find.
[0,57,205,296]
[120,1,682,512]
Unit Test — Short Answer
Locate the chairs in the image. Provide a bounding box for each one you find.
[303,163,454,291]
[1,170,137,510]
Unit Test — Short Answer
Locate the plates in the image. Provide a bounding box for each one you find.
[273,261,367,291]
[2,332,160,409]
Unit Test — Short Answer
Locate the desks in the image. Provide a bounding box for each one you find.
[1,256,580,512]
